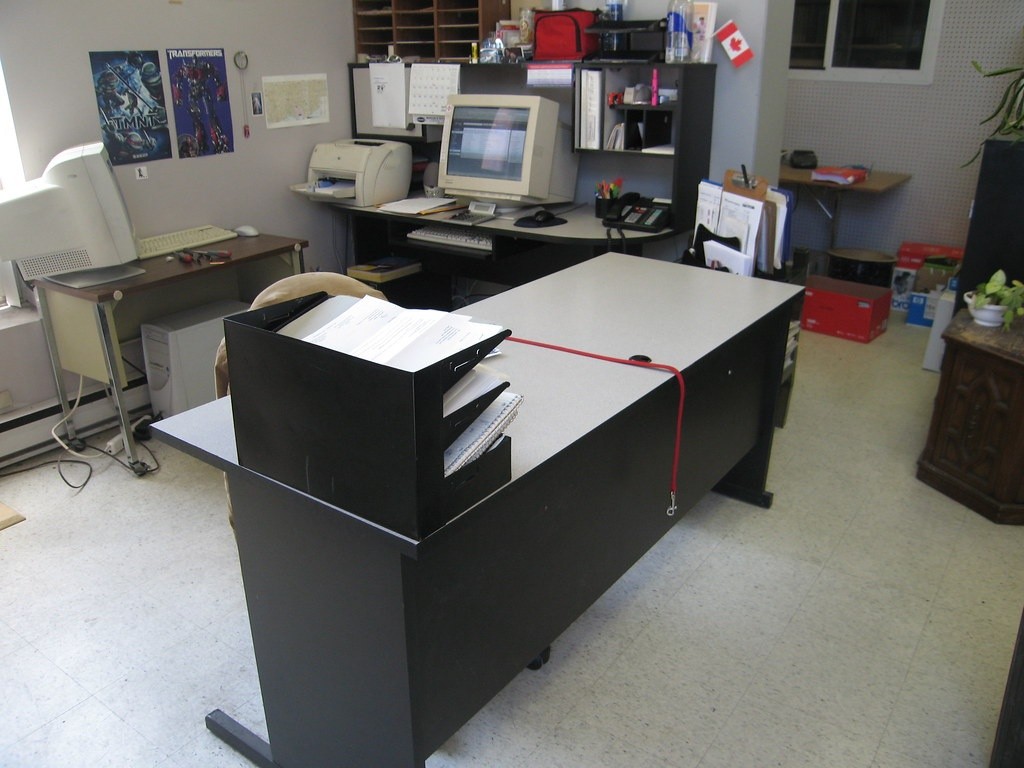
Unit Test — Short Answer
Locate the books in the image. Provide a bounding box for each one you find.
[444,392,524,477]
[607,123,644,150]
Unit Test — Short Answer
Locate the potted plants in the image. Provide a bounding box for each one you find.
[962,268,1024,332]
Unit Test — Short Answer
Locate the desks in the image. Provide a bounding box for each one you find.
[146,254,806,768]
[33,234,311,477]
[340,192,691,305]
[777,163,913,251]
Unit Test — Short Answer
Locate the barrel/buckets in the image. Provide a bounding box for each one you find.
[827,247,899,289]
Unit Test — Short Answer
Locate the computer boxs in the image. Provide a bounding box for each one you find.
[141,300,255,420]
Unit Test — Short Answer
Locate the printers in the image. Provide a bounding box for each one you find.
[290,138,412,206]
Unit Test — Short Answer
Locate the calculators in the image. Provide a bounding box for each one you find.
[443,201,496,227]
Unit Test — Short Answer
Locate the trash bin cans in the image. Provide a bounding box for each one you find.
[347,260,421,309]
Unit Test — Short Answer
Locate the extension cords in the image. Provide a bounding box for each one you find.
[108,415,152,455]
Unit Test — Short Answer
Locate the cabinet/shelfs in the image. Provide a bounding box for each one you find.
[914,306,1024,525]
[345,0,720,220]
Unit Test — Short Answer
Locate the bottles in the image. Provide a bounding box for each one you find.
[601,0,628,59]
[664,0,695,64]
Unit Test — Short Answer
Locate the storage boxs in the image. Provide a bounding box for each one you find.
[888,240,966,311]
[800,271,893,345]
[904,291,945,328]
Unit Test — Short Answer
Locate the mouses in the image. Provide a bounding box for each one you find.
[535,210,555,222]
[235,225,259,237]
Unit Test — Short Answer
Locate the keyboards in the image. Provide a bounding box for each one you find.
[136,224,238,260]
[406,224,492,251]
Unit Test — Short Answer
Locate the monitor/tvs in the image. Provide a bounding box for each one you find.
[437,95,580,219]
[0,141,146,289]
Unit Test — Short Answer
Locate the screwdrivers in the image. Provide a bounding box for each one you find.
[174,252,192,262]
[194,249,232,258]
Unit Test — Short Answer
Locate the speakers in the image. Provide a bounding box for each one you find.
[423,162,445,199]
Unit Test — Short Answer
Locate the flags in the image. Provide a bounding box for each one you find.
[717,22,753,67]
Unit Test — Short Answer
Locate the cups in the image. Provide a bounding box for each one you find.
[596,197,617,218]
[518,6,536,45]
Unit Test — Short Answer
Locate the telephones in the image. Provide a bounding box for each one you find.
[602,191,671,233]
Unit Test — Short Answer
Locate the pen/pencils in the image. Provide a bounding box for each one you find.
[594,176,622,199]
[740,164,750,189]
